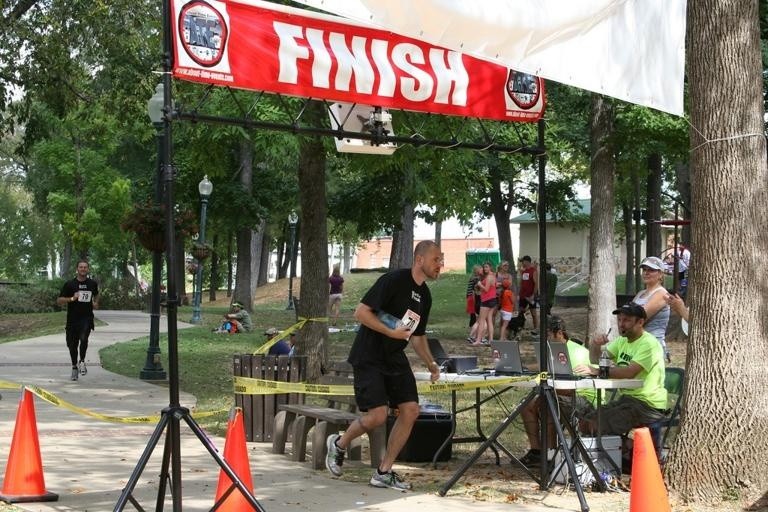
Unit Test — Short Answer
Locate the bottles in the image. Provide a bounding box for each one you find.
[599,345,610,378]
[589,471,612,483]
[380,313,408,331]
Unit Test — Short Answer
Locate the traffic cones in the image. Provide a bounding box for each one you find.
[1,385,60,504]
[629,426,671,511]
[212,404,258,511]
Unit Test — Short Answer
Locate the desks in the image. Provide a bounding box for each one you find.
[405,368,644,488]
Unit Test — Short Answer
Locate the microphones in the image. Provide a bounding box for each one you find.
[622,322,637,334]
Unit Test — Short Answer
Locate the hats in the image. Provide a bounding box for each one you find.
[518,256,531,262]
[531,315,566,335]
[503,280,512,288]
[612,301,647,319]
[232,301,244,309]
[264,328,297,336]
[638,256,665,270]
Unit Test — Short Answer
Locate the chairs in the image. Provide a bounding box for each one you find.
[624,360,686,462]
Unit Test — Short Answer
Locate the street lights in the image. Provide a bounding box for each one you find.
[131,85,178,382]
[284,209,299,310]
[189,174,214,326]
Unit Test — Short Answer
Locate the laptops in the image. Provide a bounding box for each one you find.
[490,340,539,377]
[533,341,597,379]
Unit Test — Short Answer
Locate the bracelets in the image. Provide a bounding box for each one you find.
[70,296,73,303]
[426,360,436,368]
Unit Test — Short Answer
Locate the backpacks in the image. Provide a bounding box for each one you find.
[227,320,237,335]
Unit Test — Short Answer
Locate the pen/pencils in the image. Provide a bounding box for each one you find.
[606,328,611,339]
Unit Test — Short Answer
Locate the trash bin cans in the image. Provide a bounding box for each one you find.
[233,353,307,442]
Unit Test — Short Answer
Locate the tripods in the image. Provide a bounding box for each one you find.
[112,0,262,510]
[438,121,606,510]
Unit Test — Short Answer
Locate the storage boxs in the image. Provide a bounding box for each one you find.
[384,410,454,464]
[552,432,627,487]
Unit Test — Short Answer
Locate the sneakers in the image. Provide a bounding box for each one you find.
[332,319,337,326]
[370,464,411,491]
[79,361,86,376]
[325,434,347,476]
[71,365,78,381]
[510,449,542,467]
[466,336,493,347]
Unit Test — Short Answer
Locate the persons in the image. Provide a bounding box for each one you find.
[661,289,689,338]
[627,255,671,356]
[509,313,588,469]
[328,267,344,327]
[462,252,559,347]
[323,239,445,490]
[262,326,289,367]
[664,240,690,280]
[285,328,298,365]
[211,300,252,334]
[55,259,100,382]
[577,301,670,477]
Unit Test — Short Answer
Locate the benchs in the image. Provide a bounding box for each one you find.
[274,356,365,464]
